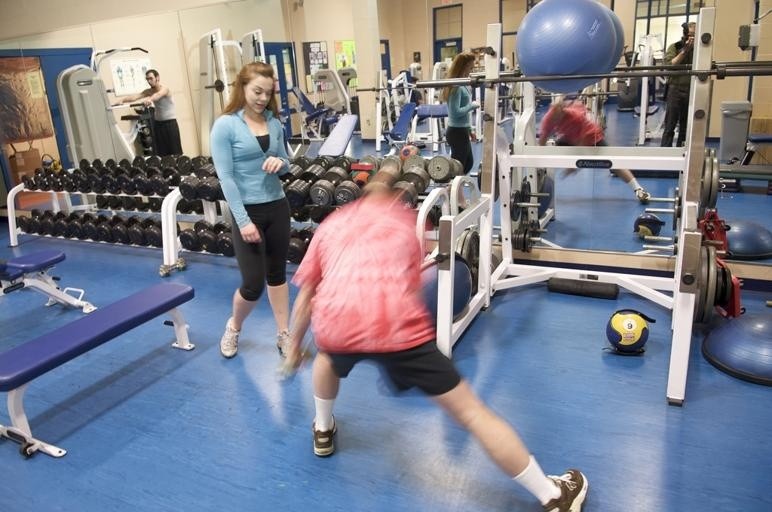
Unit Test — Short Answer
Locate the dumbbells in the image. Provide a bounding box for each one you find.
[17,168,235,264]
[291,156,464,183]
[73,157,213,174]
[287,227,316,263]
[281,164,440,231]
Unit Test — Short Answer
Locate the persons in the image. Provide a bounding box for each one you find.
[531,96,653,205]
[209,61,304,359]
[443,51,482,175]
[659,23,696,147]
[275,171,591,512]
[109,70,184,157]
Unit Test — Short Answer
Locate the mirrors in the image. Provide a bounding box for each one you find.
[0,0,772,279]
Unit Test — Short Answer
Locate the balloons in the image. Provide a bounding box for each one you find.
[589,4,625,87]
[515,1,614,94]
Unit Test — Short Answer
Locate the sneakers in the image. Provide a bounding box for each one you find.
[542,468,589,512]
[313,414,338,458]
[220,317,241,358]
[277,332,290,355]
[636,190,650,202]
